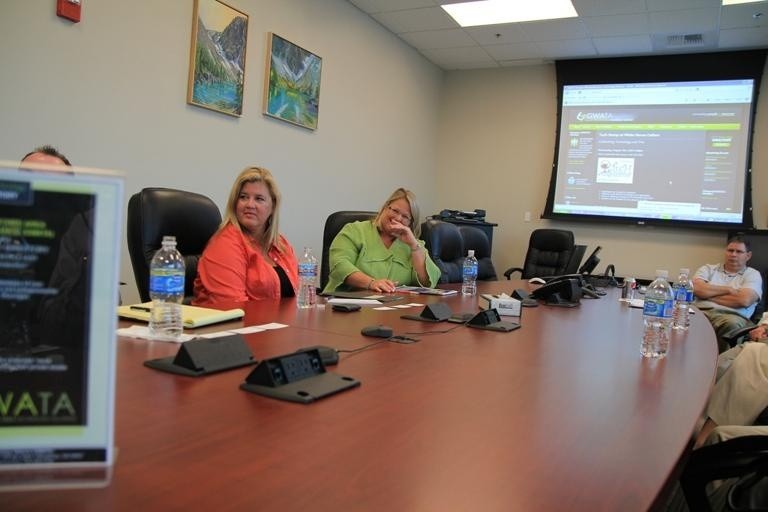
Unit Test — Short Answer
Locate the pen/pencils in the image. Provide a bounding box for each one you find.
[130,306,150,312]
[119,283,127,285]
[395,289,419,295]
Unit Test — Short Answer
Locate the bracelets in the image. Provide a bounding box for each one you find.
[411,244,420,251]
[367,278,374,290]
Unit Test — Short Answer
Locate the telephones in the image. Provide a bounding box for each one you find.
[532,274,585,304]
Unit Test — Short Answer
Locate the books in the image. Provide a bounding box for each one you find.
[117,300,245,329]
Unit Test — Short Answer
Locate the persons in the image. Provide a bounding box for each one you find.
[189,167,300,305]
[693,236,768,495]
[18,145,73,175]
[322,188,441,295]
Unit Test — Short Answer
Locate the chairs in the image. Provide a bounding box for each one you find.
[319,209,382,289]
[681,433,767,511]
[723,326,760,347]
[505,226,576,278]
[422,219,465,283]
[127,185,223,307]
[458,225,497,282]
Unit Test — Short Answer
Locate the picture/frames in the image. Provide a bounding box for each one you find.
[185,0,250,122]
[261,29,324,132]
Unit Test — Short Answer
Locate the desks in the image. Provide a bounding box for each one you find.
[1,271,722,510]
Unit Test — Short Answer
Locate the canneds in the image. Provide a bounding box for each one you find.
[622,277,636,299]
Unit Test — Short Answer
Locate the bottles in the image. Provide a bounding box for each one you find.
[296,245,320,309]
[461,249,480,296]
[146,235,187,339]
[671,267,693,330]
[639,269,674,361]
